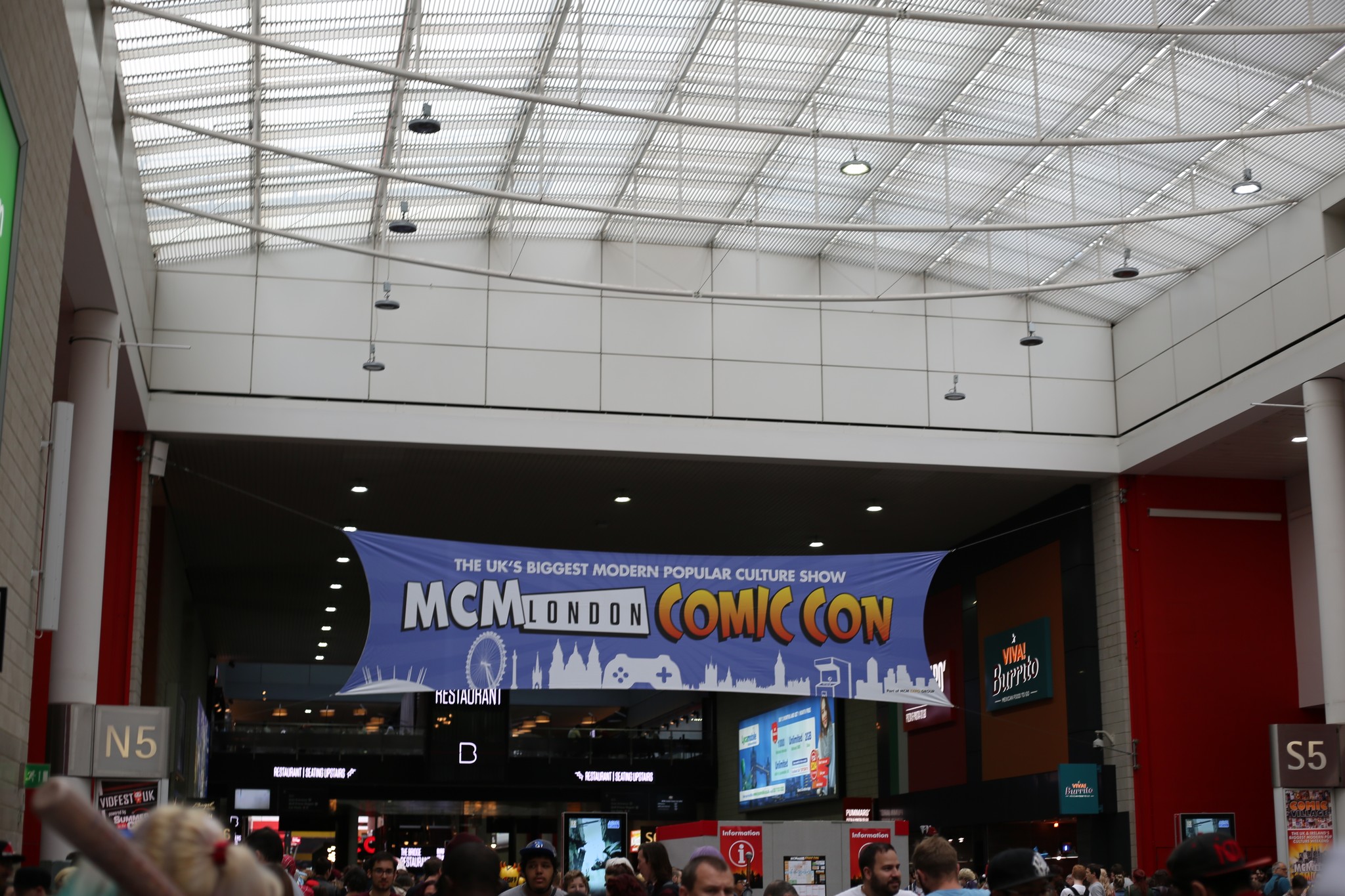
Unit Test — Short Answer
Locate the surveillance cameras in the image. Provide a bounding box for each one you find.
[1093,739,1104,751]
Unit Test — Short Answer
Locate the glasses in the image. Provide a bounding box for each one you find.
[1277,867,1288,871]
[737,880,746,885]
[372,868,394,877]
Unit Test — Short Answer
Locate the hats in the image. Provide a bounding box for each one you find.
[441,830,484,868]
[1165,832,1274,884]
[519,839,557,862]
[986,849,1060,895]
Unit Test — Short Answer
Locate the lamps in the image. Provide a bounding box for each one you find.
[363,343,385,371]
[406,76,441,136]
[1019,292,1044,346]
[838,133,870,177]
[944,374,965,401]
[1229,136,1261,193]
[386,179,417,234]
[375,281,399,310]
[1113,246,1138,279]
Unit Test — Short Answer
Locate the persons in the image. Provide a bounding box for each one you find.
[19,753,1315,894]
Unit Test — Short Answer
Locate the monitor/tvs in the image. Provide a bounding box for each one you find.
[737,696,845,813]
[1174,812,1237,848]
[562,812,628,896]
